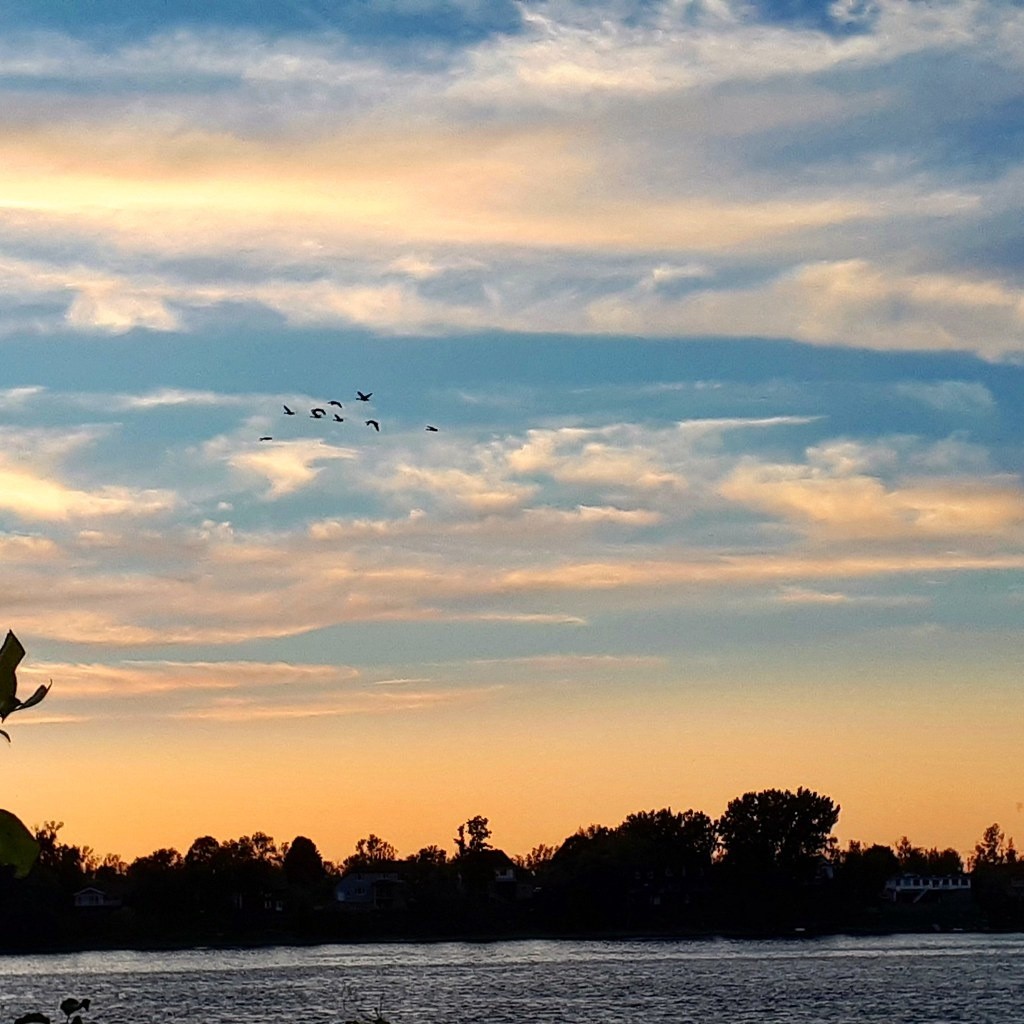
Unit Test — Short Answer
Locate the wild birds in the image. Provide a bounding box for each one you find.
[327,400,343,408]
[424,424,440,432]
[260,436,273,441]
[332,413,344,422]
[59,998,91,1024]
[283,405,295,416]
[366,420,380,432]
[356,391,373,401]
[310,408,326,418]
